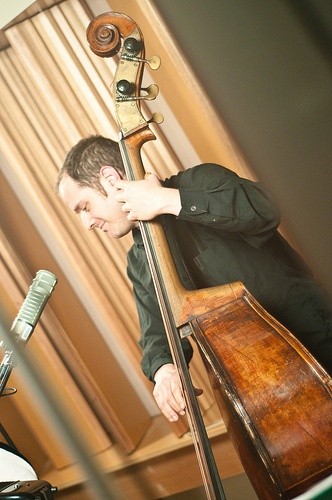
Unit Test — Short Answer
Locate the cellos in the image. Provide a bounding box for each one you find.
[86,10,332,500]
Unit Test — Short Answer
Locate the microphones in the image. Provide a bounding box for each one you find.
[1,268,59,362]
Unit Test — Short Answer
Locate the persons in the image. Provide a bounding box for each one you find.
[55,137,332,422]
